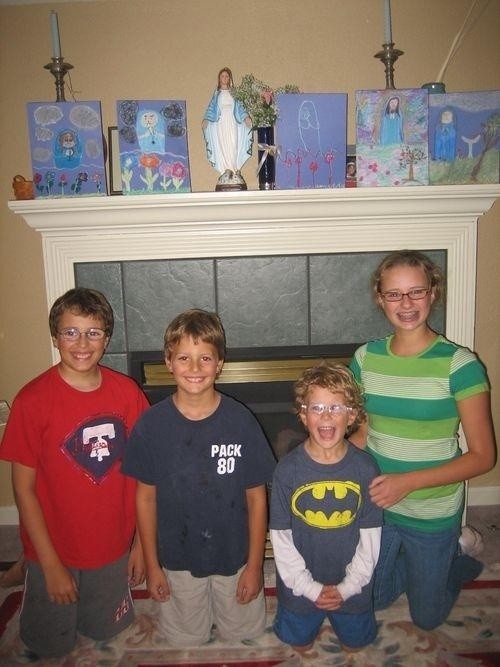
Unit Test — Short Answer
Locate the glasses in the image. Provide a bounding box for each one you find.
[300,402,353,414]
[55,327,108,341]
[380,279,432,302]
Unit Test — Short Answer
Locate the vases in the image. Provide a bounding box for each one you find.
[257,127,275,190]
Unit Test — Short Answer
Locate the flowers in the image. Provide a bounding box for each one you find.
[229,73,301,135]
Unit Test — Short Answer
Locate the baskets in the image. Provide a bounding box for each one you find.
[12,175,34,200]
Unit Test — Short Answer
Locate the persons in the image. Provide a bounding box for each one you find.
[346,249,497,632]
[118,307,280,647]
[198,66,255,181]
[0,285,152,660]
[267,359,384,650]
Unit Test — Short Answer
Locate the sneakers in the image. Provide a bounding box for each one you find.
[458,525,485,558]
[0,551,26,588]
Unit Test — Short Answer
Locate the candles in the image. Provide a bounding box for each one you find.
[384,0,391,43]
[51,12,60,57]
